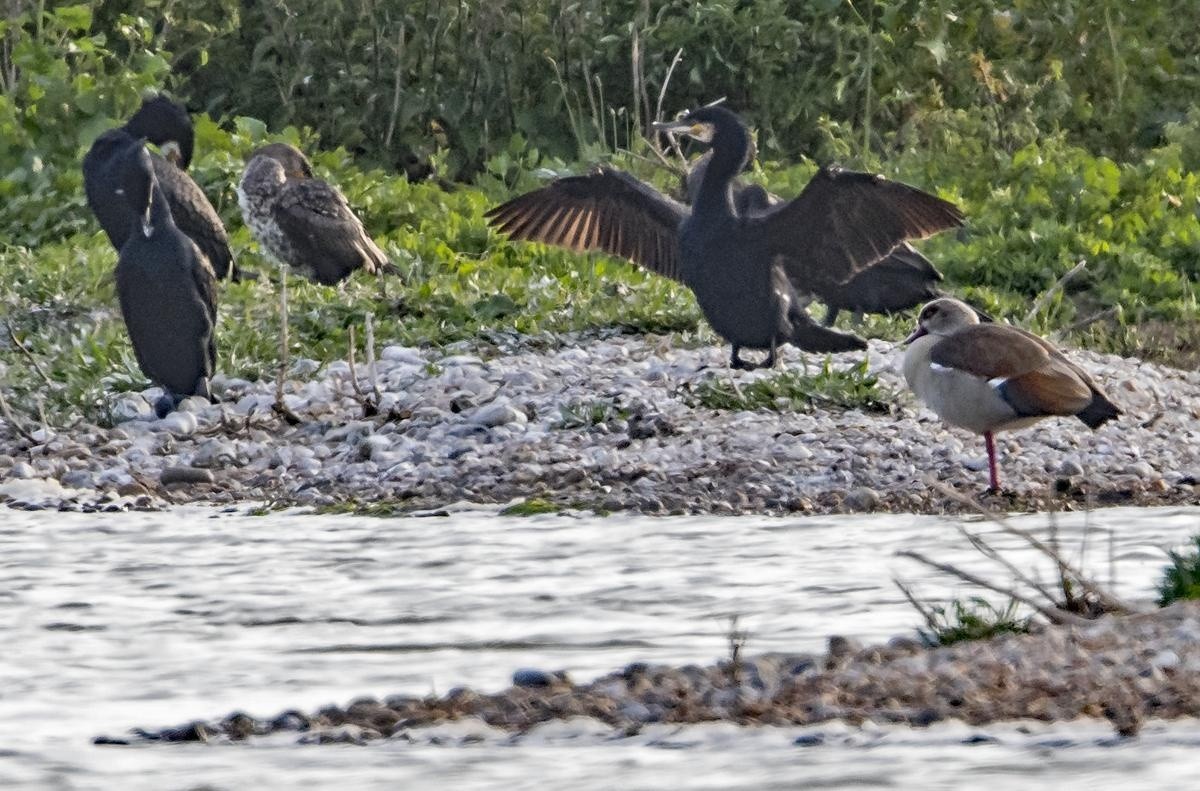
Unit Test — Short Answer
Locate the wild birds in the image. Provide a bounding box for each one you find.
[479,105,996,374]
[238,144,404,408]
[901,296,1126,505]
[81,93,280,421]
